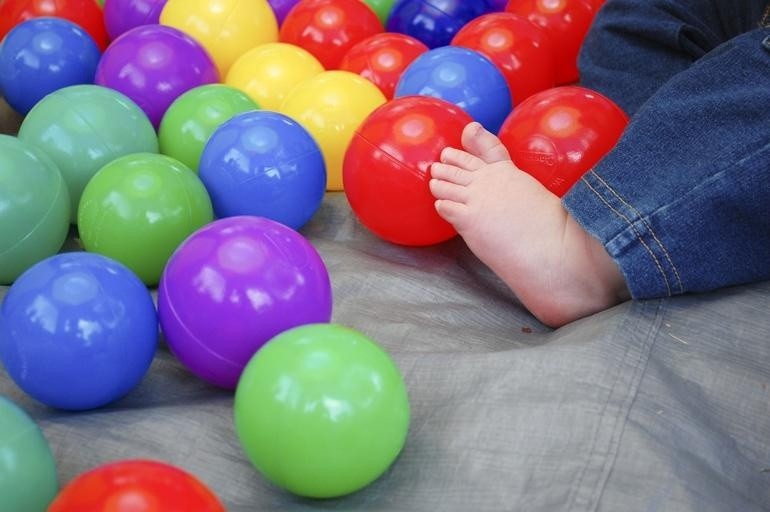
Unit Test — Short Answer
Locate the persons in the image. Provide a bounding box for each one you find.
[429,0,770,328]
